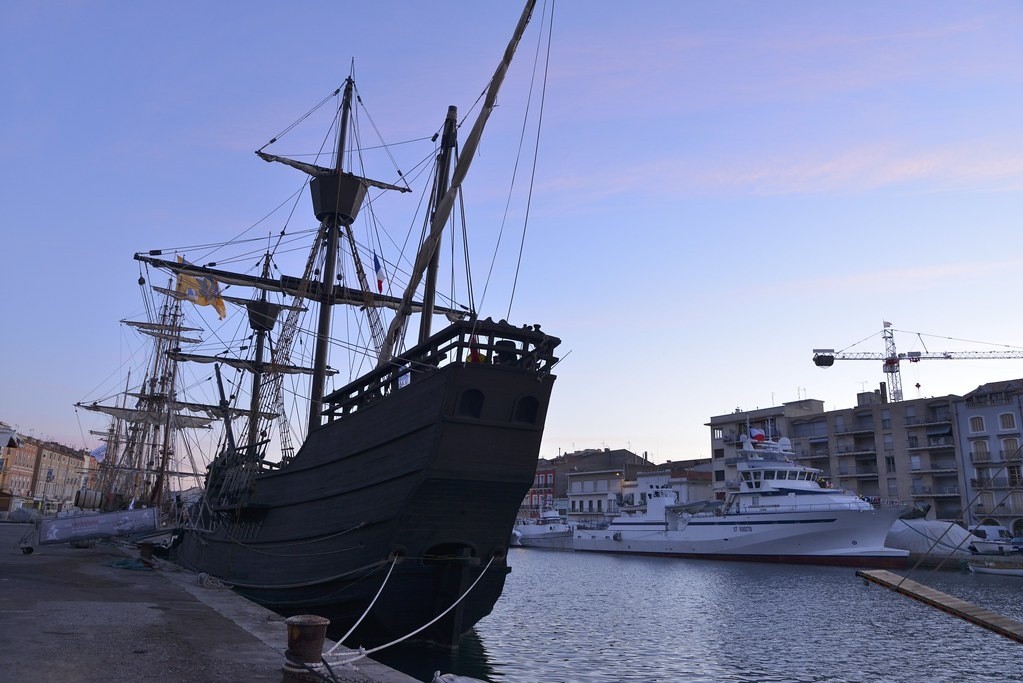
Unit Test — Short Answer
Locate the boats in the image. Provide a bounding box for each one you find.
[509,497,572,550]
[966,562,1023,576]
[572,406,911,568]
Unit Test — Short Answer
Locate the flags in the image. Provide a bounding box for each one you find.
[92,445,118,464]
[174,255,226,320]
[374,250,385,294]
[749,428,765,442]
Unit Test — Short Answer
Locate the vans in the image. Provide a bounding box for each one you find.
[969,525,1013,541]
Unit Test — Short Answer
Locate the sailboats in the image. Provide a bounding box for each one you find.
[74,0,573,646]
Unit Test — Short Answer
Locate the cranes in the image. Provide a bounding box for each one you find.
[811,320,1023,403]
